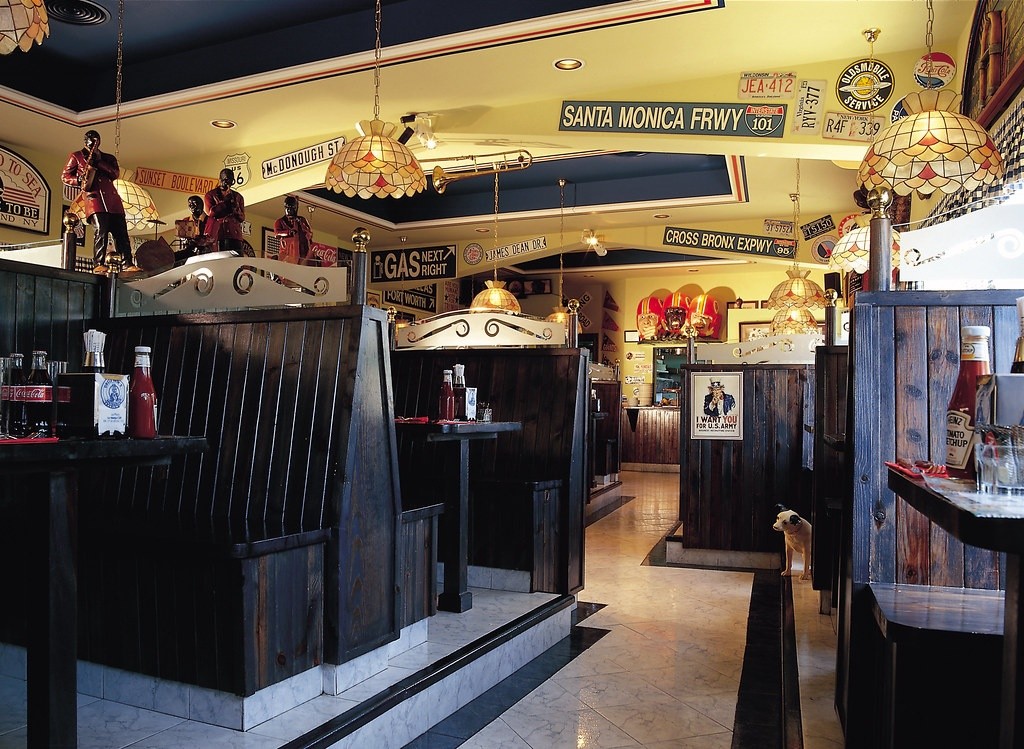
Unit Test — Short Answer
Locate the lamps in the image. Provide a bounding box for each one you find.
[766,2,1006,332]
[70,0,159,230]
[413,114,439,150]
[0,0,50,56]
[325,0,428,198]
[544,179,582,335]
[468,161,521,315]
[580,229,607,257]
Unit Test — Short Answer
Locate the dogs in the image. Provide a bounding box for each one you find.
[772,504,812,580]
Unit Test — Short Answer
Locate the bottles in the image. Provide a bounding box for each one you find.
[439,370,455,421]
[452,375,467,420]
[0,350,54,438]
[129,345,157,440]
[1010,336,1024,374]
[947,326,996,480]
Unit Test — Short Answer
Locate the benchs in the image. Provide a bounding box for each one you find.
[813,288,1024,749]
[0,307,624,695]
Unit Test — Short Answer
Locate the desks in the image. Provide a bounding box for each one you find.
[889,465,1024,748]
[398,418,522,615]
[0,436,211,746]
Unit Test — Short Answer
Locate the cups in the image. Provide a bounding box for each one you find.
[973,445,1024,496]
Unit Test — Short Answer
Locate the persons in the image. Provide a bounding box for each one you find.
[181,168,246,258]
[635,292,722,340]
[703,377,736,417]
[273,196,313,264]
[61,130,143,275]
